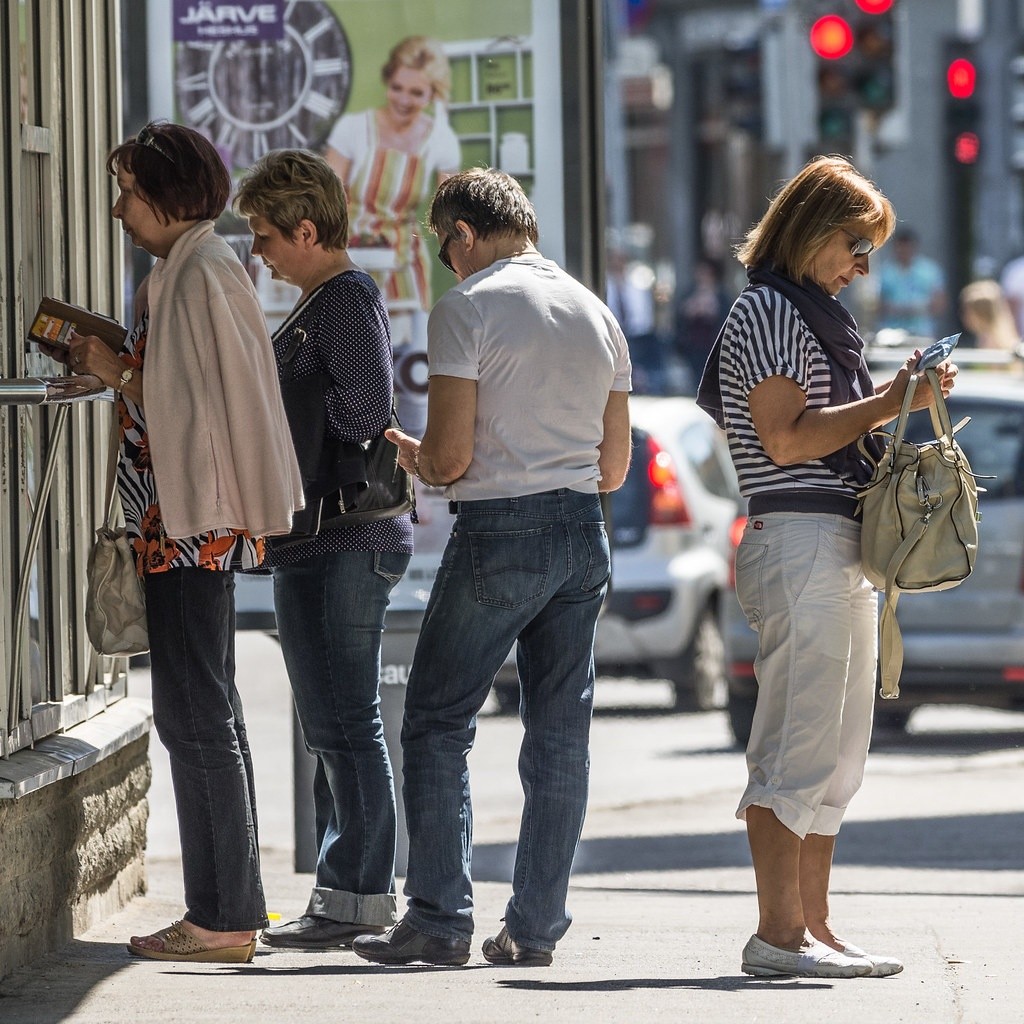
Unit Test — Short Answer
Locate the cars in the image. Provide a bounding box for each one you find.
[492,349,1022,751]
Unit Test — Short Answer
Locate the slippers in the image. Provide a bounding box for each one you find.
[127,911,259,962]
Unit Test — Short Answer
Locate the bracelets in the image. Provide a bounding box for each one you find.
[414,462,433,488]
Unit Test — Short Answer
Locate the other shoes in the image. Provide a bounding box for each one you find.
[741,930,872,977]
[837,939,904,977]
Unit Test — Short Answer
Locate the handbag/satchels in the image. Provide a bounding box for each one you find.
[860,369,998,594]
[84,531,152,658]
[279,284,419,531]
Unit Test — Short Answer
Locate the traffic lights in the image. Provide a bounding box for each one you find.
[940,41,983,178]
[811,1,854,150]
[850,0,897,110]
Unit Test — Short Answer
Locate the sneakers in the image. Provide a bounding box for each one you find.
[481,915,553,967]
[353,914,468,966]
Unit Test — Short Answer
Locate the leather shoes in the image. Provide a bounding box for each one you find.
[261,913,386,949]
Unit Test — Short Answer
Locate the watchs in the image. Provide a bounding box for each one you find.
[177,1,354,174]
[117,367,135,393]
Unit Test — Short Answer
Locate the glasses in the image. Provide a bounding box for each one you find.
[830,219,877,258]
[137,126,177,162]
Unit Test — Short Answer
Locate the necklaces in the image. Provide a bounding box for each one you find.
[501,251,538,257]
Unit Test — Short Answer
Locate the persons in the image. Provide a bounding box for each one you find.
[322,33,461,313]
[38,125,305,965]
[878,227,1024,373]
[232,149,412,949]
[354,169,632,966]
[696,158,959,979]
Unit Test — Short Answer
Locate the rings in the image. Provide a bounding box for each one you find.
[75,353,80,364]
